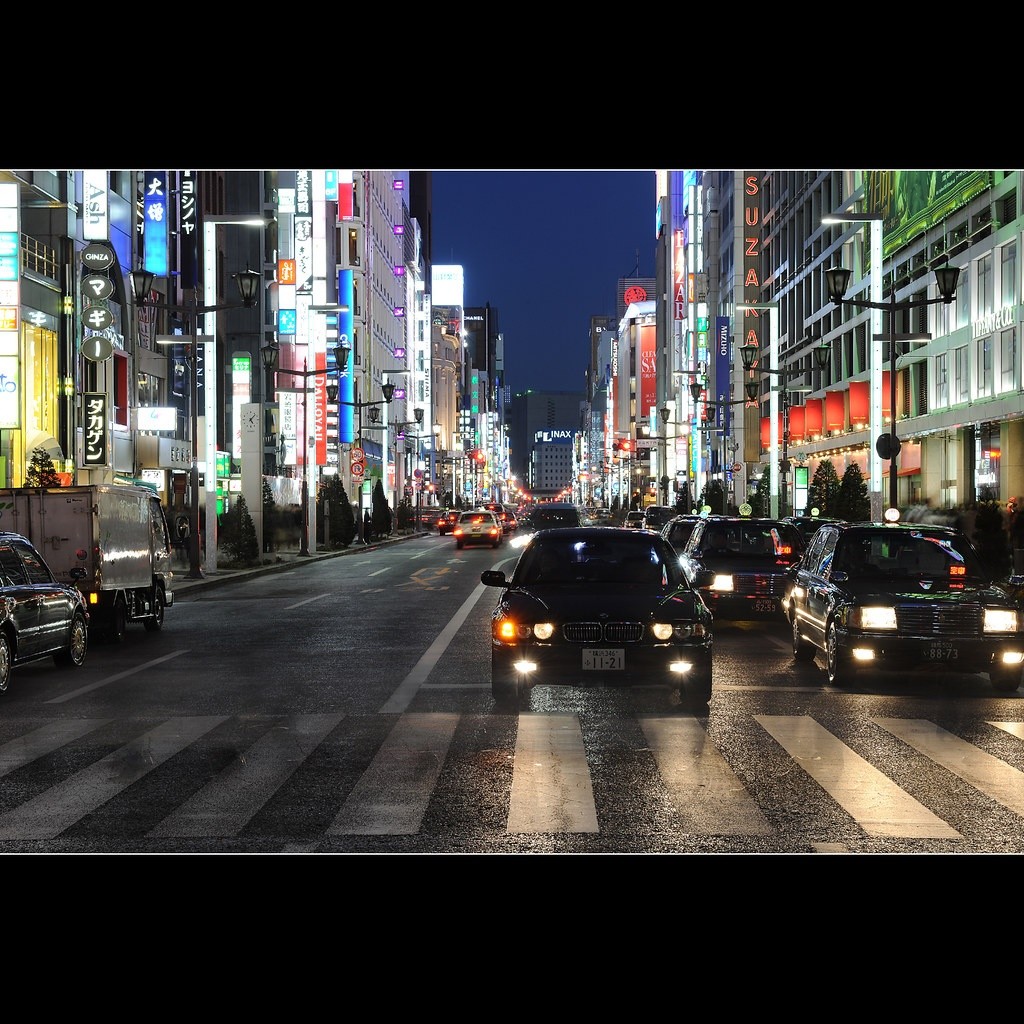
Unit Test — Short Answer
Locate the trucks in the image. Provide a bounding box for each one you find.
[0,483,192,646]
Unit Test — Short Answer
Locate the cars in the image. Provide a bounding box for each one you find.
[681,513,810,618]
[480,526,717,706]
[413,500,851,570]
[777,521,1023,685]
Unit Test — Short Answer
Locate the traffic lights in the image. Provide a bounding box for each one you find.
[612,444,629,451]
[468,453,484,460]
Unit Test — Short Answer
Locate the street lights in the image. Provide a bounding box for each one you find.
[824,253,961,521]
[259,339,351,561]
[368,404,425,540]
[817,210,885,559]
[398,419,442,532]
[692,378,762,518]
[738,338,832,518]
[598,457,610,511]
[131,259,265,579]
[659,403,716,517]
[324,378,397,546]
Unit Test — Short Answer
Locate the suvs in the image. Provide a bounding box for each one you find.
[0,529,91,700]
